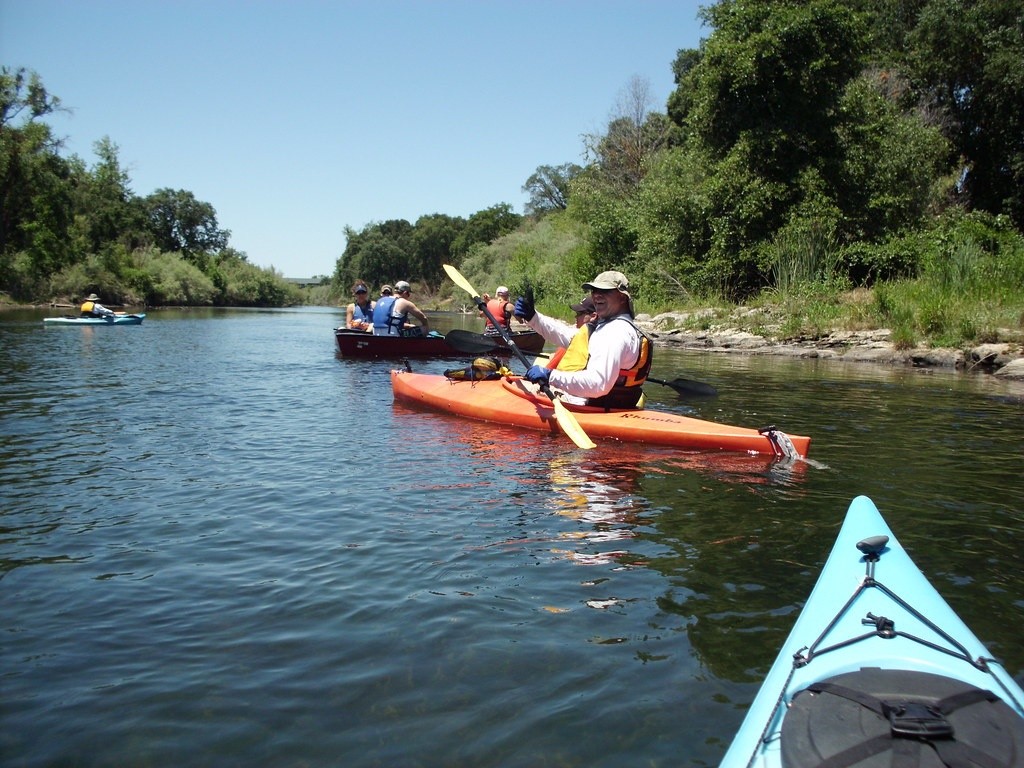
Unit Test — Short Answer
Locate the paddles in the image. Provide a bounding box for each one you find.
[445,328,719,395]
[440,261,595,449]
[116,314,139,318]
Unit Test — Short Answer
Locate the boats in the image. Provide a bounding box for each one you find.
[43,313,146,325]
[391,365,812,461]
[716,496,1023,768]
[334,327,546,356]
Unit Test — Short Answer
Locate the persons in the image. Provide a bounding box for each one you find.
[80,294,115,317]
[546,298,599,372]
[513,271,653,412]
[481,286,524,337]
[373,281,429,337]
[381,285,393,296]
[346,280,376,333]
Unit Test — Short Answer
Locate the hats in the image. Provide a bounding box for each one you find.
[394,281,415,293]
[354,285,367,293]
[569,295,595,313]
[86,293,101,301]
[582,271,629,291]
[496,286,511,296]
[381,284,392,293]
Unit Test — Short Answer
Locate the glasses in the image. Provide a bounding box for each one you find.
[356,290,367,295]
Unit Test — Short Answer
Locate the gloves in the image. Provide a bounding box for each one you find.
[514,287,536,322]
[525,365,553,385]
